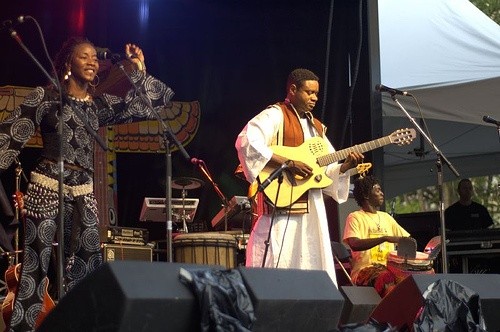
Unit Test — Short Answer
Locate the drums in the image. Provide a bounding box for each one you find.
[386,249,435,283]
[172,231,240,271]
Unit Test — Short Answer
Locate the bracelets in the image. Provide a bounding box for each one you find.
[340,170,345,173]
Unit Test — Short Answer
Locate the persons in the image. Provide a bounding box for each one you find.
[343,174,411,297]
[444,179,495,242]
[235,68,365,291]
[0,37,174,332]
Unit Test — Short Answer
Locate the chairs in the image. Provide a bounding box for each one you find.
[332,241,351,286]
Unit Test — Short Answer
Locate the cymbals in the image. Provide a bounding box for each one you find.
[165,176,204,190]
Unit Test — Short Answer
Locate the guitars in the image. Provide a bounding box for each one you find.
[256,127,416,210]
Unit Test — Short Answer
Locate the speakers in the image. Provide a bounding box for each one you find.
[34,245,500,332]
[211,196,252,234]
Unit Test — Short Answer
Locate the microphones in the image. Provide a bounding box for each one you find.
[97,50,137,61]
[0,16,30,31]
[375,81,411,97]
[482,115,500,127]
[257,160,291,192]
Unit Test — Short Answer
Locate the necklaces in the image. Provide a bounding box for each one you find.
[68,94,89,101]
